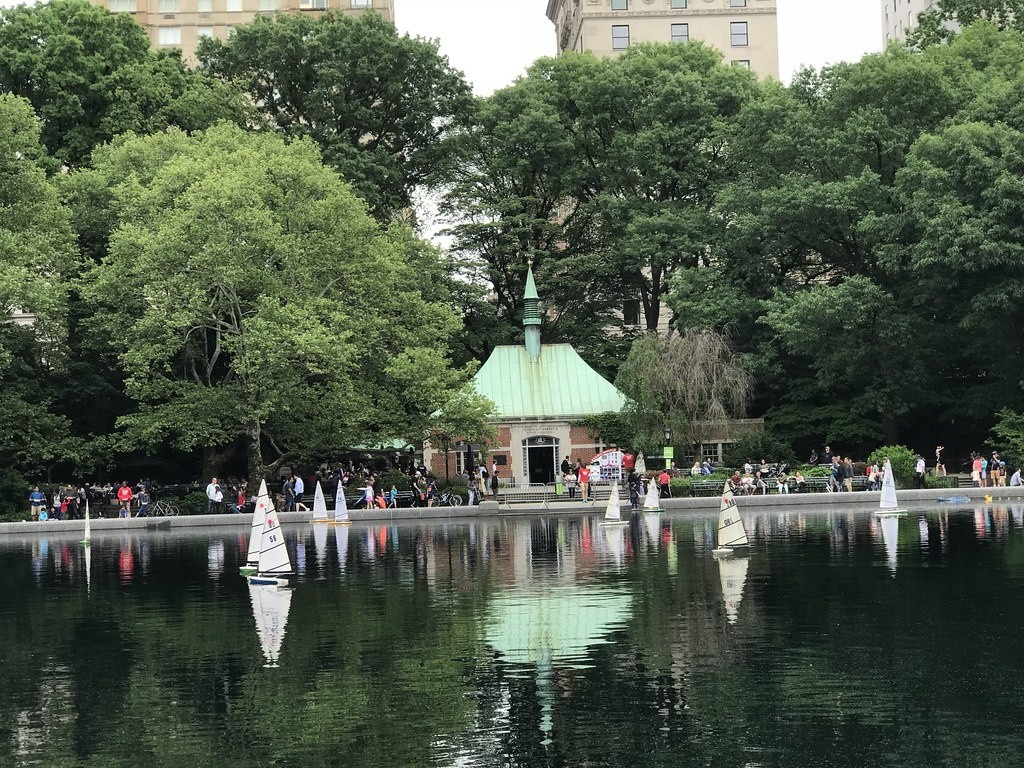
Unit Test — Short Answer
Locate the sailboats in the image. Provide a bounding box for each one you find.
[84,545,92,599]
[873,459,909,517]
[331,524,352,573]
[312,522,328,572]
[328,479,353,525]
[308,480,335,523]
[715,558,750,624]
[879,516,902,577]
[604,525,629,572]
[642,477,667,512]
[644,512,661,553]
[597,481,629,526]
[249,586,293,670]
[244,499,295,587]
[711,480,748,558]
[80,500,93,545]
[238,478,269,575]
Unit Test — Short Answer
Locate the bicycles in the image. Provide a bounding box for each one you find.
[432,485,462,506]
[148,499,180,516]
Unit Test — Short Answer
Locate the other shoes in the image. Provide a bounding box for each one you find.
[306,508,310,511]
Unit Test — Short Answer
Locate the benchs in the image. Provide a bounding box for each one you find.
[225,500,251,513]
[690,476,871,497]
[299,491,415,508]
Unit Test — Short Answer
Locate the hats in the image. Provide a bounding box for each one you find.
[992,451,997,453]
[416,471,421,474]
[703,462,707,464]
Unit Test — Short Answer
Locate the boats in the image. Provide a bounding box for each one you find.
[938,494,970,502]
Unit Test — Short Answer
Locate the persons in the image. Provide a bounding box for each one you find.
[28,476,161,521]
[204,471,310,515]
[561,446,1024,510]
[325,459,500,509]
